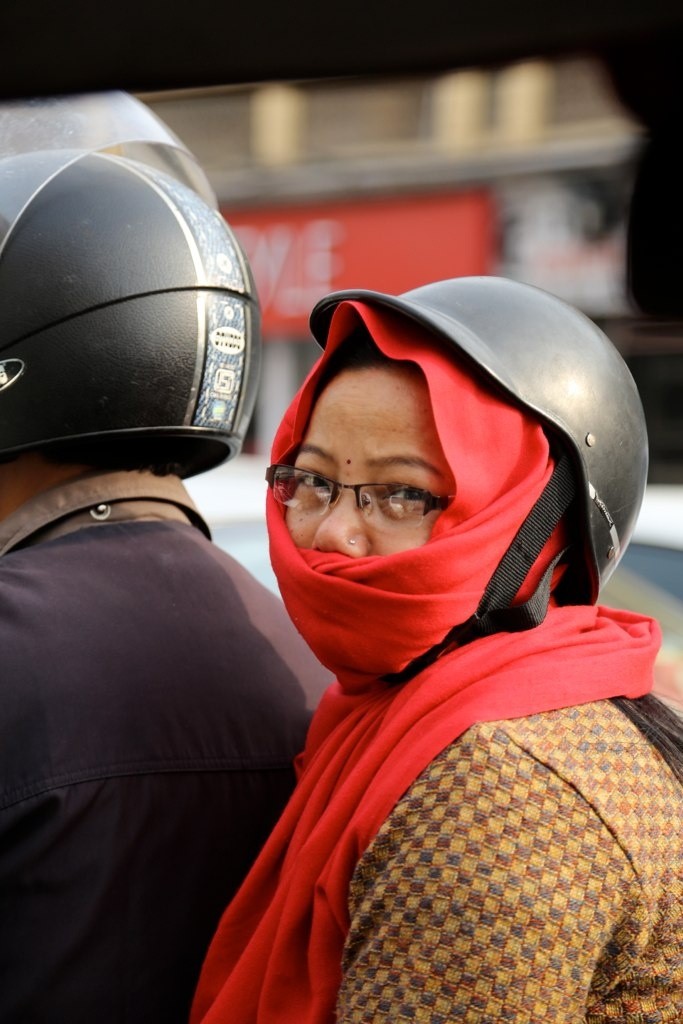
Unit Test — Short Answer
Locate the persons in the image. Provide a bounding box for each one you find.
[267,276,683,1024]
[0,151,337,1024]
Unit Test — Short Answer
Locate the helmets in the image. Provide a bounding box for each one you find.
[1,151,262,481]
[307,275,649,607]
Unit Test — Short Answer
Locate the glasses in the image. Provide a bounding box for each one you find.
[264,464,456,531]
